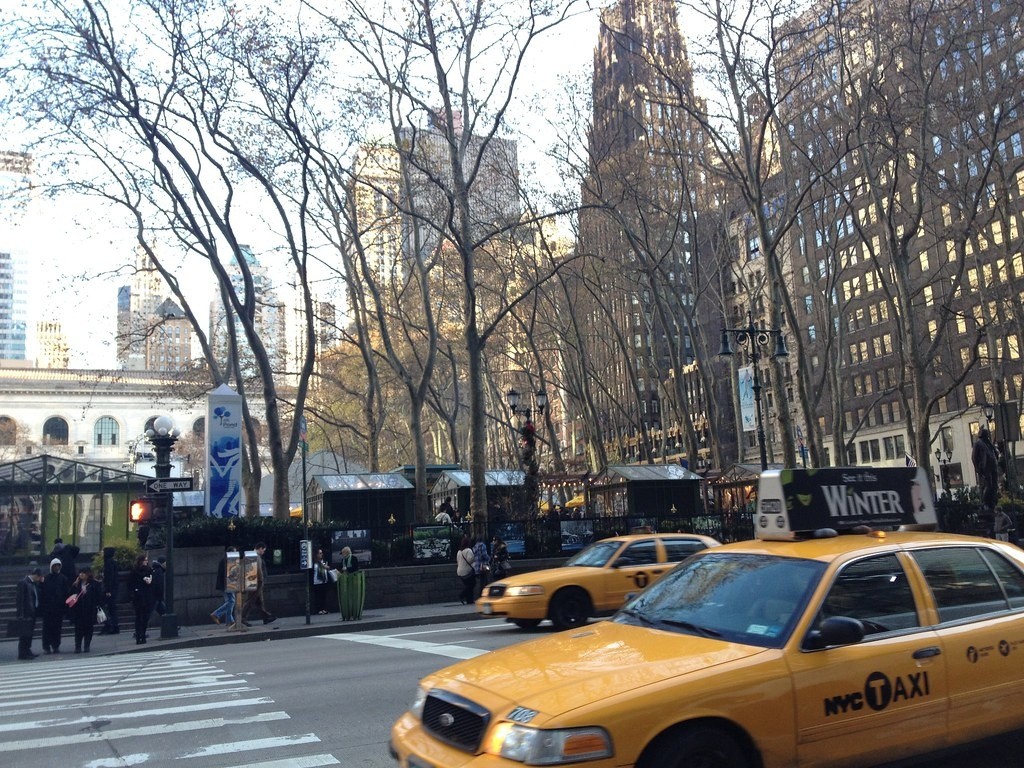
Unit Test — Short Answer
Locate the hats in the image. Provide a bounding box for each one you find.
[995,506,1002,511]
[461,538,470,546]
[158,556,167,564]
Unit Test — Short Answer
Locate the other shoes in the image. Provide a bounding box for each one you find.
[319,610,328,615]
[210,613,220,625]
[54,648,60,653]
[263,617,277,625]
[74,646,81,653]
[136,635,146,644]
[84,646,91,653]
[44,649,52,654]
[18,652,39,659]
[226,621,236,626]
[242,618,252,627]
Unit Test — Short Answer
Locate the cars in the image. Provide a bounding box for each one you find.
[387,528,1024,768]
[476,532,740,632]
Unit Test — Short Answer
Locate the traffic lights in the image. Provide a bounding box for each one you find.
[129,500,152,521]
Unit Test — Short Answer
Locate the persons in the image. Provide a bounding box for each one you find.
[50,538,76,580]
[16,567,42,660]
[134,555,181,637]
[209,546,236,626]
[40,558,71,654]
[73,568,112,653]
[994,506,1012,542]
[98,547,119,635]
[241,542,278,627]
[489,536,509,581]
[972,429,998,510]
[435,496,464,532]
[340,546,358,574]
[132,555,153,644]
[472,538,490,592]
[548,505,581,534]
[310,549,328,614]
[457,538,476,604]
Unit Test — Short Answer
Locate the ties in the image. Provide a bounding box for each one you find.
[34,584,38,608]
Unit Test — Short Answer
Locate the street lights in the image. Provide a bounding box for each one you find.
[935,448,952,498]
[507,388,548,559]
[145,416,181,640]
[718,309,790,471]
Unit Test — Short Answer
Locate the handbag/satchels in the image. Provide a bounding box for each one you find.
[6,618,33,638]
[328,568,343,582]
[65,593,79,608]
[501,561,512,570]
[472,567,479,577]
[96,606,107,624]
[479,562,492,574]
[59,544,80,561]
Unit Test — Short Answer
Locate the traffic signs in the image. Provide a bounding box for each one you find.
[146,477,193,495]
